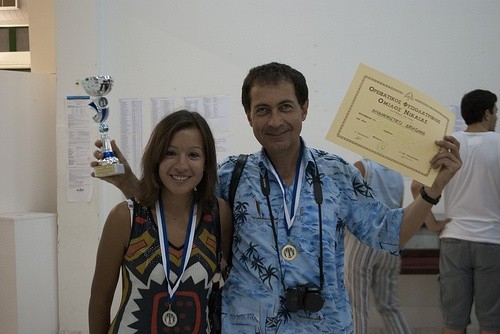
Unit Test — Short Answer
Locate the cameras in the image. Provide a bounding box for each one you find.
[284,281,325,313]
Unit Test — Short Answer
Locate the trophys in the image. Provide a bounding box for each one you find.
[80,74,126,177]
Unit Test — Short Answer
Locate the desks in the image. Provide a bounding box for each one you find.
[400,210,447,274]
[0,211,58,334]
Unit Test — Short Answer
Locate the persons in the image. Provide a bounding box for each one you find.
[89,63,462,334]
[340,158,408,334]
[409,88,500,334]
[89,110,234,334]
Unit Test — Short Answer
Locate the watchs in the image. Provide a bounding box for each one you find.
[419,186,442,206]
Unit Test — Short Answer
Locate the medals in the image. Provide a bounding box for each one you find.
[281,246,297,260]
[162,310,177,327]
